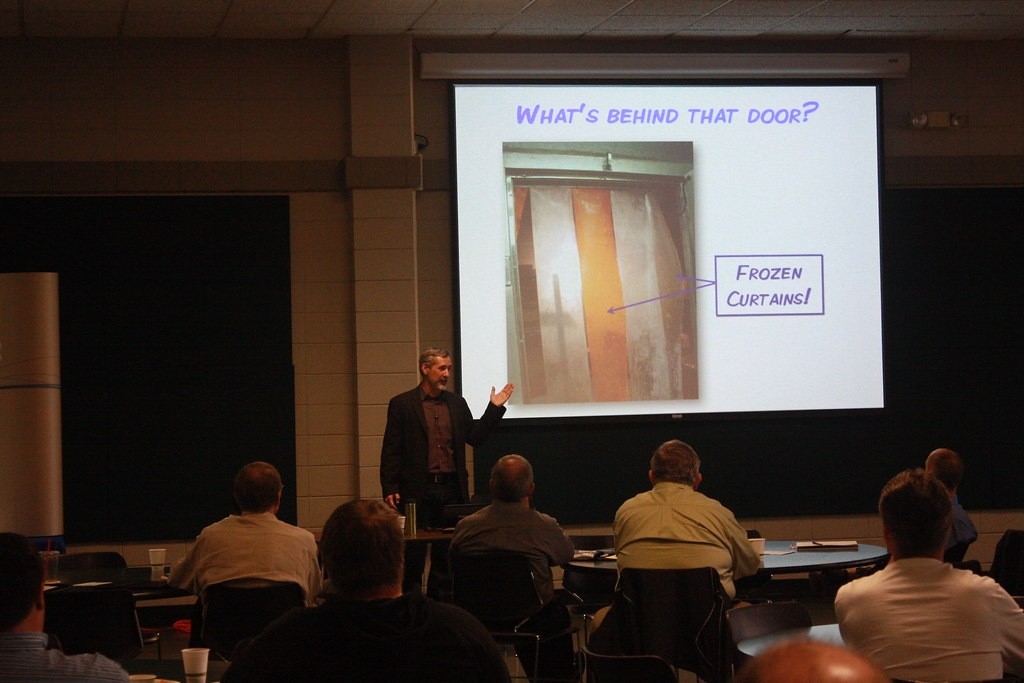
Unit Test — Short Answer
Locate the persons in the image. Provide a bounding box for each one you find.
[733,641,887,683]
[0,532,130,683]
[169,460,321,662]
[856,448,969,575]
[587,439,761,683]
[448,455,575,683]
[379,346,515,598]
[220,498,512,683]
[834,468,1024,683]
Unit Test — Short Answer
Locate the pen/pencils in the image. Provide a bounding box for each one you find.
[813,541,822,545]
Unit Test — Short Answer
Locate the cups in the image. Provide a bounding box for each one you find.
[750,538,765,561]
[148,548,166,566]
[129,673,157,683]
[181,647,210,683]
[397,516,406,534]
[39,550,60,583]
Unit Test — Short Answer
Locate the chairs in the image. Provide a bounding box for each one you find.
[196,576,307,662]
[60,552,130,579]
[47,589,144,669]
[450,547,813,683]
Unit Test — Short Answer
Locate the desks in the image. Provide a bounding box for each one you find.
[561,538,890,590]
[738,622,844,660]
[44,565,199,621]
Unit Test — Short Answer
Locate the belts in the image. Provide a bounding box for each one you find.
[427,474,459,483]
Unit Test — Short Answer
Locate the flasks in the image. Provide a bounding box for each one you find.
[405,498,416,537]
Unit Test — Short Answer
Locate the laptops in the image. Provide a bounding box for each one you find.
[424,503,488,532]
